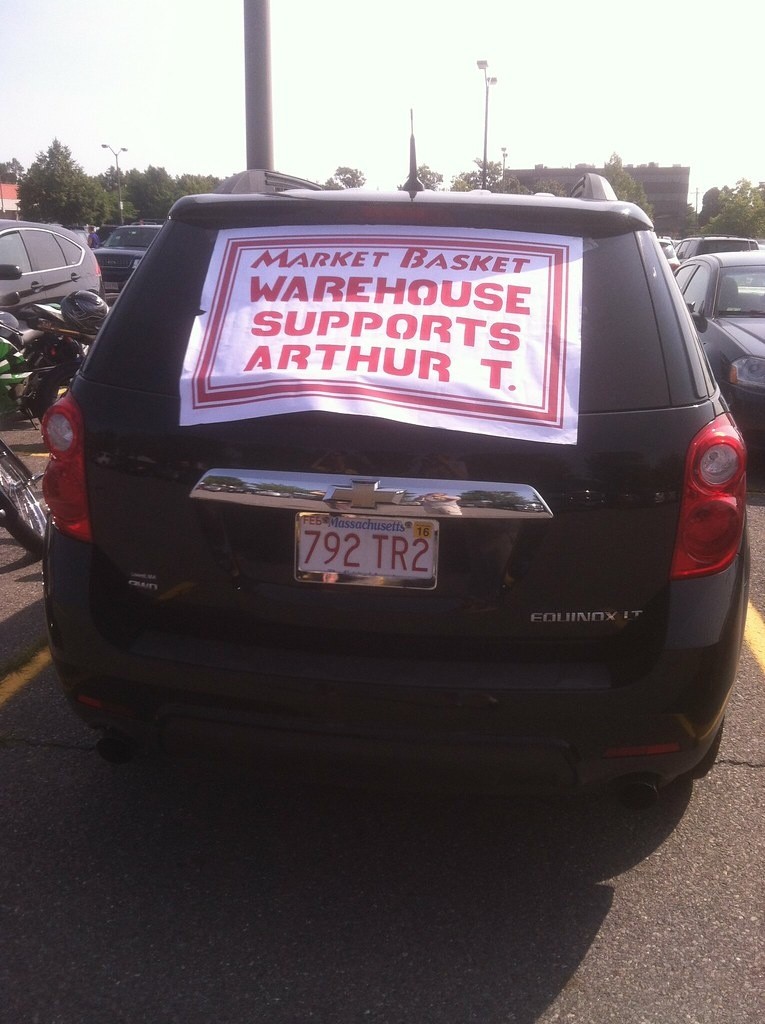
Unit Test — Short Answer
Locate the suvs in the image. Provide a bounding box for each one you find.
[42,169,751,812]
[0,217,106,325]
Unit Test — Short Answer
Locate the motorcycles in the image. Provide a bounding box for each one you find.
[1,264,110,557]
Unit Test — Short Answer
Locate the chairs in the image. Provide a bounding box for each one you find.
[718,277,739,311]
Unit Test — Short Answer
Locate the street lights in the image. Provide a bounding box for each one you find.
[501,147,508,193]
[691,188,701,237]
[100,144,128,225]
[476,60,496,190]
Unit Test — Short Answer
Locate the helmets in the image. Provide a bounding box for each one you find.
[61,290,109,335]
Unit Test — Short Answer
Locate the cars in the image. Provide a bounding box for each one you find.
[672,248,765,457]
[657,233,765,273]
[50,223,120,249]
[92,223,164,293]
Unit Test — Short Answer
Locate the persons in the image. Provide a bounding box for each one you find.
[87,227,101,250]
[139,218,146,225]
